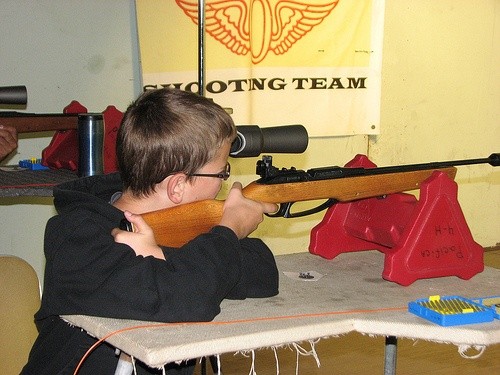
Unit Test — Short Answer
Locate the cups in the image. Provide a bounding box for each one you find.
[79,114,105,177]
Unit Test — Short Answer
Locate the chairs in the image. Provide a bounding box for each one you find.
[0,255,42,375]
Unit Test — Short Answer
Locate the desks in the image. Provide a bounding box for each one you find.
[0,167,78,197]
[60,251,500,375]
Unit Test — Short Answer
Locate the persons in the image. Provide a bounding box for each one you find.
[19,89,280,375]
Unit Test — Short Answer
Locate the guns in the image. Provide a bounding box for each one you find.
[0,86,102,133]
[120,153,500,256]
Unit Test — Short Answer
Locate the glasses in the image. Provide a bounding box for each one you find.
[155,162,231,184]
